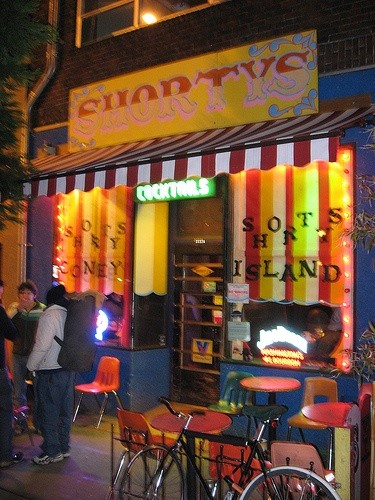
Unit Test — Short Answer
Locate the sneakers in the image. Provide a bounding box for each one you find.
[61,448,71,458]
[33,452,64,466]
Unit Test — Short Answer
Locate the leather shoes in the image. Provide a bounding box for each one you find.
[0,452,23,467]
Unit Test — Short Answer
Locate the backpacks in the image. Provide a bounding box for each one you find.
[49,291,108,371]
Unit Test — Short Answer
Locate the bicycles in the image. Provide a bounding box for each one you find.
[118,396,342,500]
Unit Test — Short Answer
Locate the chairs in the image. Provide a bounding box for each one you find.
[271,442,335,500]
[7,369,35,447]
[286,376,338,470]
[107,408,178,500]
[72,355,123,429]
[208,371,259,440]
[207,442,271,500]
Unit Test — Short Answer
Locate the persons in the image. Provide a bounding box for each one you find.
[10,279,47,436]
[1,280,25,467]
[24,283,78,466]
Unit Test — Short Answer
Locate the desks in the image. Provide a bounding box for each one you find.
[239,375,302,440]
[149,409,233,500]
[302,401,359,470]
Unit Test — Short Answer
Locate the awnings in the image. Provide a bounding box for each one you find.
[15,103,374,199]
